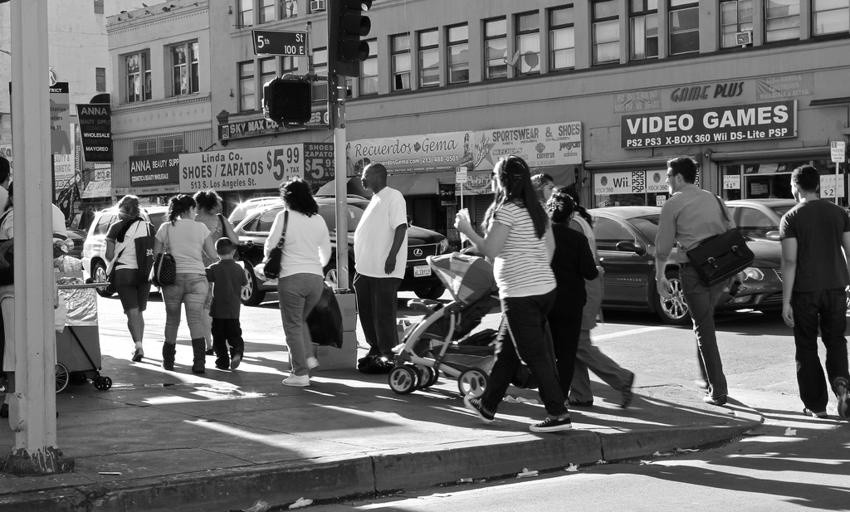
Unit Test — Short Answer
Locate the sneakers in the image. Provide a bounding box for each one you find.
[530,415,573,432]
[282,373,311,387]
[131,348,143,362]
[801,407,827,418]
[0,402,9,417]
[306,357,320,371]
[358,352,378,365]
[833,378,850,421]
[360,356,397,375]
[568,394,594,406]
[205,348,214,357]
[620,371,634,408]
[464,395,494,424]
[230,353,243,369]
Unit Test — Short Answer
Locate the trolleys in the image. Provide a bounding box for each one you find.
[56,280,113,392]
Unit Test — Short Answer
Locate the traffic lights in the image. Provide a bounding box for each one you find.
[327,0,372,81]
[264,78,312,126]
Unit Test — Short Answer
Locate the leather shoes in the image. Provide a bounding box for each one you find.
[702,394,727,406]
[695,380,710,393]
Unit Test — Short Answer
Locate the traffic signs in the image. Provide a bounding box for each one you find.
[252,28,313,59]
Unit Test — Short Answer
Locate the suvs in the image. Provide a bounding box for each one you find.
[81,206,170,297]
[227,194,370,230]
[230,200,451,307]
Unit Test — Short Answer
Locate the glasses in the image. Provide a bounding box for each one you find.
[666,174,674,178]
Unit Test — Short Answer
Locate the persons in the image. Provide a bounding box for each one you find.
[528,174,556,207]
[455,155,572,433]
[778,165,850,416]
[1,155,11,216]
[156,196,217,372]
[105,195,159,362]
[1,181,16,418]
[204,238,247,370]
[655,155,747,405]
[558,188,634,408]
[527,192,605,401]
[194,190,239,355]
[263,179,331,386]
[355,163,408,370]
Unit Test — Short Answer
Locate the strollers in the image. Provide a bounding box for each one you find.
[388,252,499,402]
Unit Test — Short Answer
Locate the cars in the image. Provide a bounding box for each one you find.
[582,205,784,324]
[722,198,798,240]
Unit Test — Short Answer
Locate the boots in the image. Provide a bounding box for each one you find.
[191,337,206,373]
[163,342,176,371]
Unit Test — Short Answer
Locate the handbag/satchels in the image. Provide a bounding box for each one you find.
[0,209,14,287]
[264,210,289,279]
[217,214,240,262]
[152,224,176,286]
[106,220,141,277]
[674,188,756,286]
